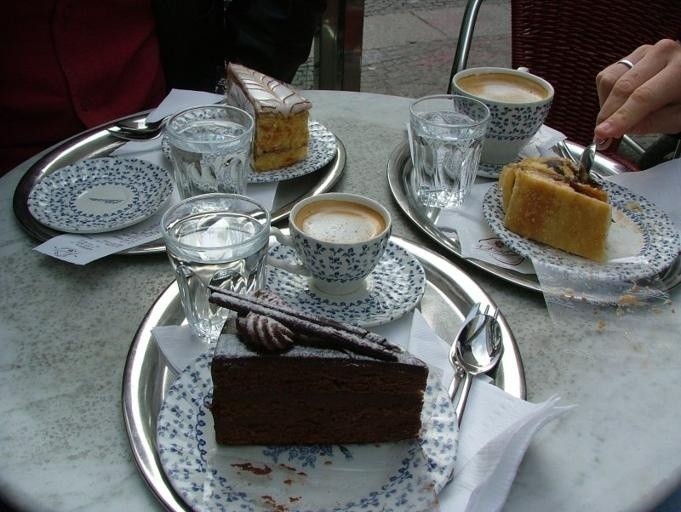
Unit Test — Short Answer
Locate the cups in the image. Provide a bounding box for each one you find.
[265,188,395,293]
[408,92,490,208]
[161,191,272,341]
[165,105,258,202]
[451,62,555,167]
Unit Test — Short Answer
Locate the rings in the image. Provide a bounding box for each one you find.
[617,57,634,69]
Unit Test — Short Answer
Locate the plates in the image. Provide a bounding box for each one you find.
[479,176,681,280]
[161,116,337,187]
[477,155,531,180]
[31,156,169,231]
[263,240,428,330]
[120,225,528,512]
[159,333,455,511]
[384,137,681,306]
[14,111,349,258]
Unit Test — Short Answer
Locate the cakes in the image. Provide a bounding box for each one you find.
[222,59,312,172]
[203,282,429,445]
[498,156,614,263]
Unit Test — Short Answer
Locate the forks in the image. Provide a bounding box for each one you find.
[105,103,229,140]
[551,141,577,164]
[446,304,500,399]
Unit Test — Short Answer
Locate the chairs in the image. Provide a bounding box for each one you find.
[444,0,681,174]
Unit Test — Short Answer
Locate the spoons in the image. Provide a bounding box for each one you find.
[116,97,230,131]
[454,314,505,430]
[577,136,599,182]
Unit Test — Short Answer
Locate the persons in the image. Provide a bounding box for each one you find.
[0,0,330,180]
[591,38,681,151]
